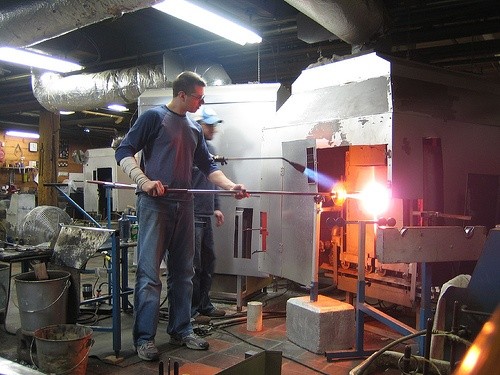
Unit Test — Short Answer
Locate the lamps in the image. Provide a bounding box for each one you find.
[0,45,86,73]
[152,0,262,46]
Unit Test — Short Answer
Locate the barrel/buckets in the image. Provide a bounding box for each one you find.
[30,324,95,375]
[12,270,72,336]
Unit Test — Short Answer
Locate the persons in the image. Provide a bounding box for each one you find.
[114,70,250,362]
[190,110,227,323]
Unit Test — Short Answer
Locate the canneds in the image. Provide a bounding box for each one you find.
[130,224,138,241]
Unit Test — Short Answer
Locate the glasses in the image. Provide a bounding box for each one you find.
[186,94,205,101]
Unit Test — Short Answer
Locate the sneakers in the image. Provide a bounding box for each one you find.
[132,341,159,360]
[170,332,209,349]
[201,309,225,317]
[192,315,211,324]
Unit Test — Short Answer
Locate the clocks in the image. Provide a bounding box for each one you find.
[29,142,38,152]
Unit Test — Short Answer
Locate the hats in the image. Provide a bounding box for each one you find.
[194,108,223,124]
